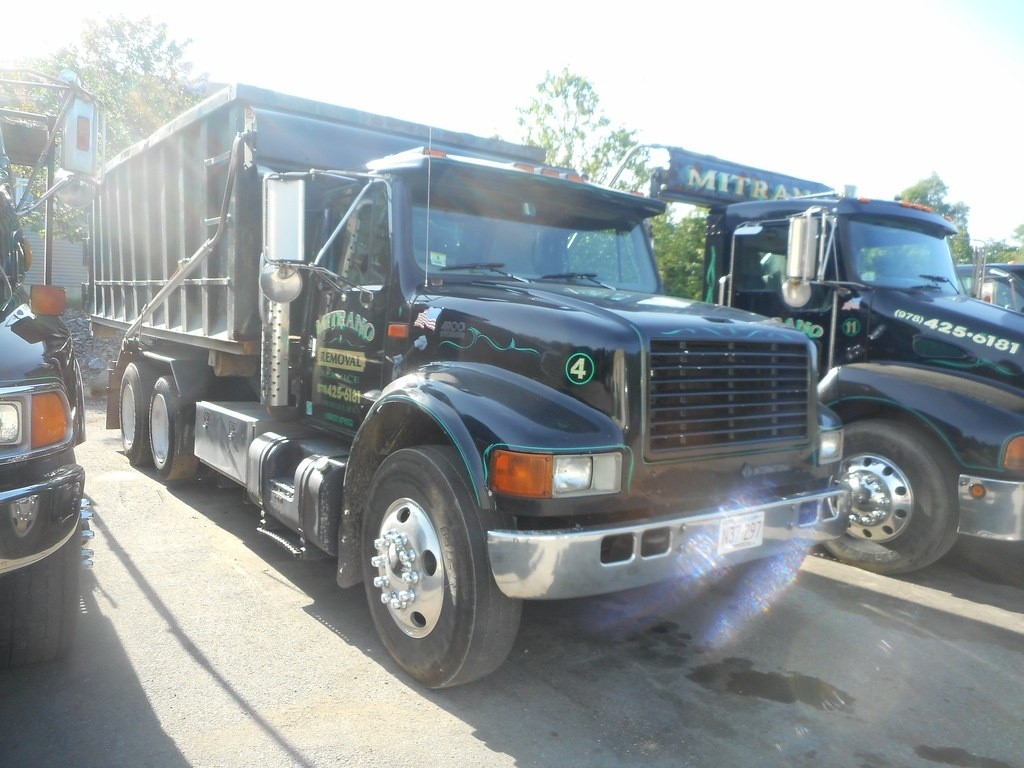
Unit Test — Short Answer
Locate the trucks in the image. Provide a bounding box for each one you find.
[82,81,853,690]
[0,123,87,682]
[571,141,1024,575]
